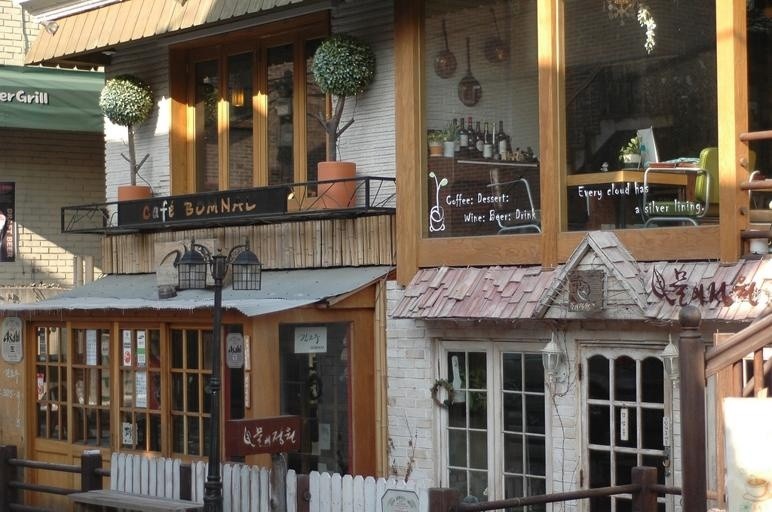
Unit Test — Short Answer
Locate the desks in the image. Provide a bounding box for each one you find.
[564,169,698,229]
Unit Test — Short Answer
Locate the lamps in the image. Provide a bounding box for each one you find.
[660,326,679,384]
[542,327,562,377]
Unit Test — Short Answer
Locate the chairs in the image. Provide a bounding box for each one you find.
[486,170,541,235]
[642,168,712,228]
[643,147,757,226]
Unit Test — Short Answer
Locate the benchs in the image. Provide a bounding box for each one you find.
[69,488,207,511]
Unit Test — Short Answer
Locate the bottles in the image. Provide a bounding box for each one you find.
[451,117,513,161]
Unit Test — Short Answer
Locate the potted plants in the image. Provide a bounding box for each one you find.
[313,30,378,213]
[100,76,154,203]
[619,137,644,169]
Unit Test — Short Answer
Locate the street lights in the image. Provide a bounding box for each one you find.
[173,234,265,512]
